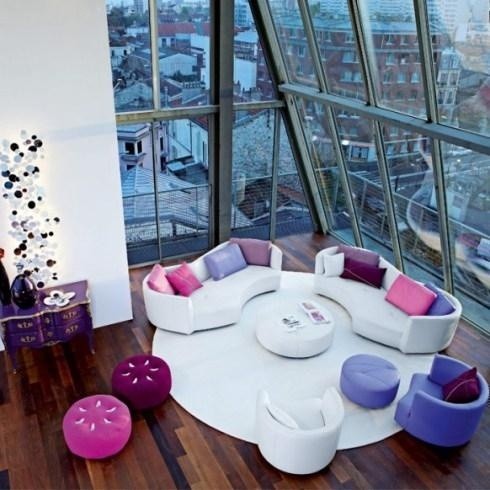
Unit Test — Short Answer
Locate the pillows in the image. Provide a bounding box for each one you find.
[443,367,479,402]
[423,282,456,315]
[231,238,272,267]
[149,265,177,296]
[167,262,203,297]
[203,243,248,281]
[341,257,387,290]
[337,245,379,266]
[324,253,344,277]
[383,274,436,316]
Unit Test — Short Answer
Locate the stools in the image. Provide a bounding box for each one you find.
[339,354,401,410]
[112,353,172,414]
[60,394,132,461]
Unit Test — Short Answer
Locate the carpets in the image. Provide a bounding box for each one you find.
[151,271,440,450]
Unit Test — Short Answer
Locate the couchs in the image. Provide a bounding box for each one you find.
[255,388,343,475]
[393,355,490,448]
[142,237,283,336]
[314,245,463,354]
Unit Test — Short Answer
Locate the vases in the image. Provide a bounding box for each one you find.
[10,274,38,309]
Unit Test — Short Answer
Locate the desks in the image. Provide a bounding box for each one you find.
[1,280,96,375]
